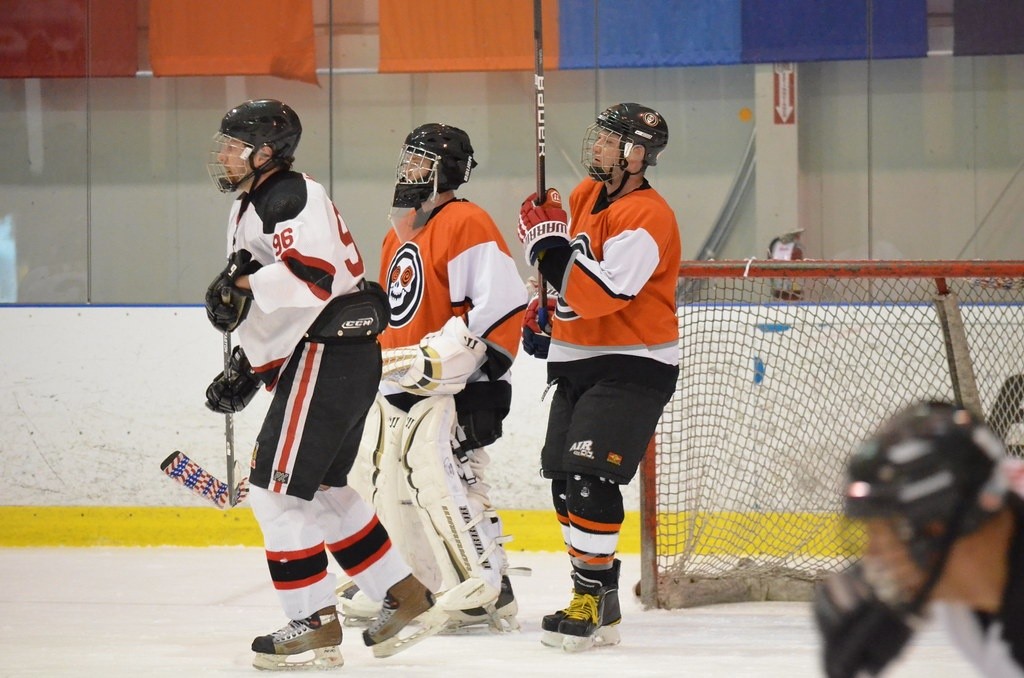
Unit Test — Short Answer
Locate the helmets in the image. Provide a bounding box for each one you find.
[219,99,302,174]
[595,102,668,167]
[842,400,1007,539]
[392,123,474,208]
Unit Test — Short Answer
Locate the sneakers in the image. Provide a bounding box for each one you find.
[435,574,523,637]
[251,605,344,672]
[361,573,452,659]
[339,585,383,627]
[541,558,622,646]
[558,573,608,655]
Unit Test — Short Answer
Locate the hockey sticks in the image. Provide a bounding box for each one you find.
[534,19,548,332]
[157,284,250,510]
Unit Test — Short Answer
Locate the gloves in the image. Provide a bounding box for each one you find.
[517,188,571,266]
[398,316,487,397]
[811,568,913,678]
[204,345,263,414]
[521,289,558,359]
[203,249,263,333]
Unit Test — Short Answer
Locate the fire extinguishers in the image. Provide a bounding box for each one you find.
[768,227,805,303]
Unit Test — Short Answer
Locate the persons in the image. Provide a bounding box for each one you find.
[809,402,1024,678]
[331,123,530,636]
[202,97,448,673]
[514,100,679,654]
